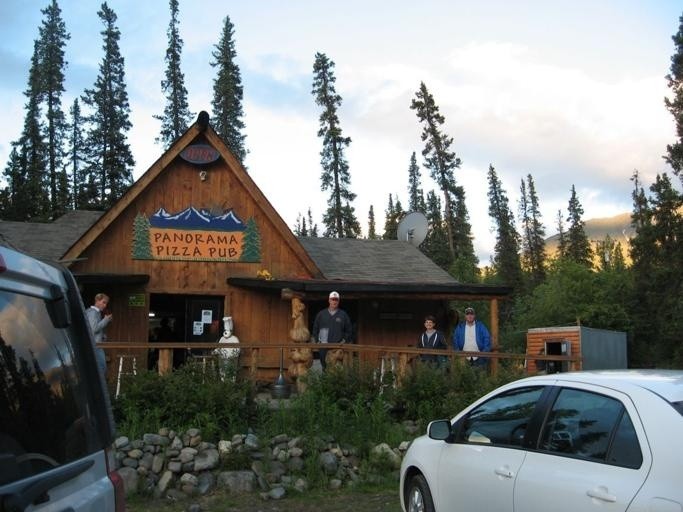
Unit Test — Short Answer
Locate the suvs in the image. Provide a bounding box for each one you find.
[0,245,128,511]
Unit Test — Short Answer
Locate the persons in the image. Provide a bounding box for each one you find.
[85,293,112,374]
[452,307,491,367]
[416,316,448,361]
[211,317,241,382]
[153,316,175,354]
[310,291,352,369]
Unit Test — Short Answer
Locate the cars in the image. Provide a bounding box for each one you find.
[392,366,682,512]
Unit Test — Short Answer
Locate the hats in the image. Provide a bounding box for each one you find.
[464,307,475,315]
[329,291,341,300]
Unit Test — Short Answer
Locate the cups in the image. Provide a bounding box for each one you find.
[103,309,112,318]
